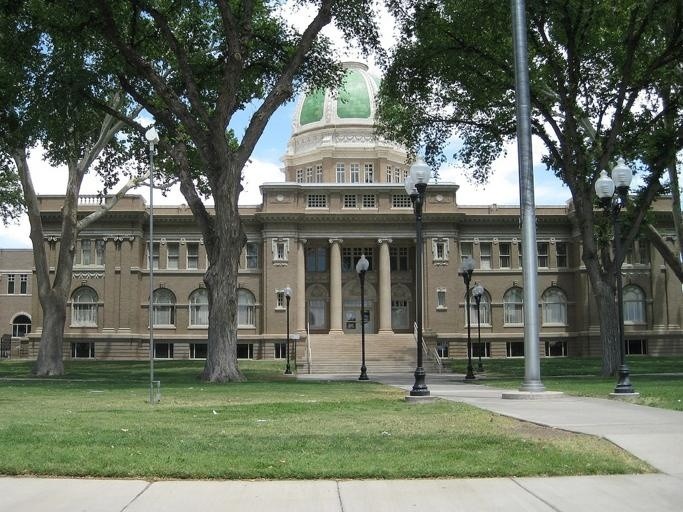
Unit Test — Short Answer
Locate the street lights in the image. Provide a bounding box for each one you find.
[594,155,635,394]
[351,252,372,381]
[470,278,487,373]
[401,150,435,398]
[457,254,478,379]
[279,284,293,376]
[142,125,162,406]
